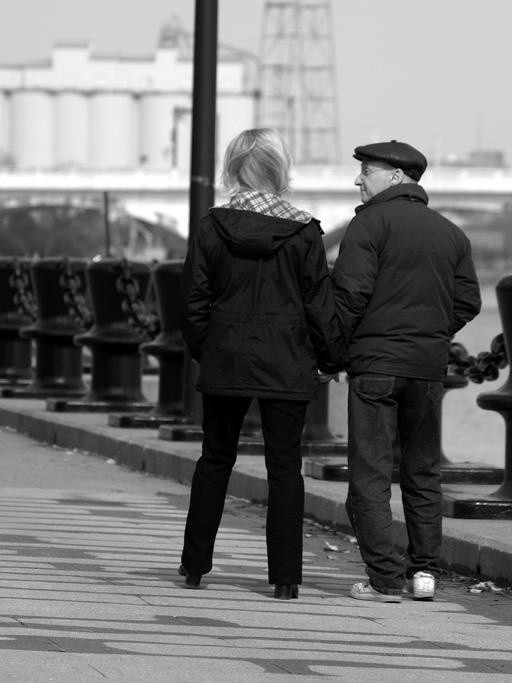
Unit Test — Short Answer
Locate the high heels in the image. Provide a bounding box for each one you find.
[178,564,201,586]
[274,582,298,600]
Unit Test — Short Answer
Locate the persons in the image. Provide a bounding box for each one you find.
[319,138,483,604]
[171,123,351,602]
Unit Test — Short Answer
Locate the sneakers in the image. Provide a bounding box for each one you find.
[402,570,436,599]
[350,582,403,603]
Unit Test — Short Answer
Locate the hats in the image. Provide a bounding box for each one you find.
[353,139,428,181]
